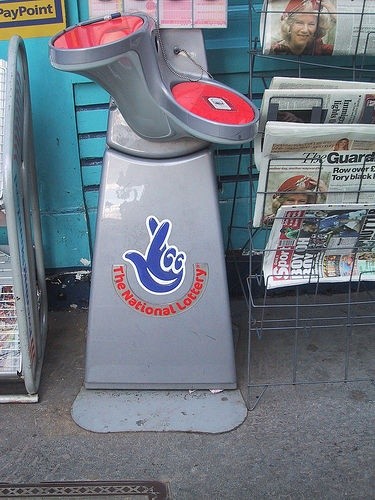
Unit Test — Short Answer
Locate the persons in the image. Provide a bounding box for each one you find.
[264,175,327,225]
[334,138,349,152]
[271,0,333,55]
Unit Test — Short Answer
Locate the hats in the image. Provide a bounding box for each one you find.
[281,0,323,20]
[272,176,316,199]
